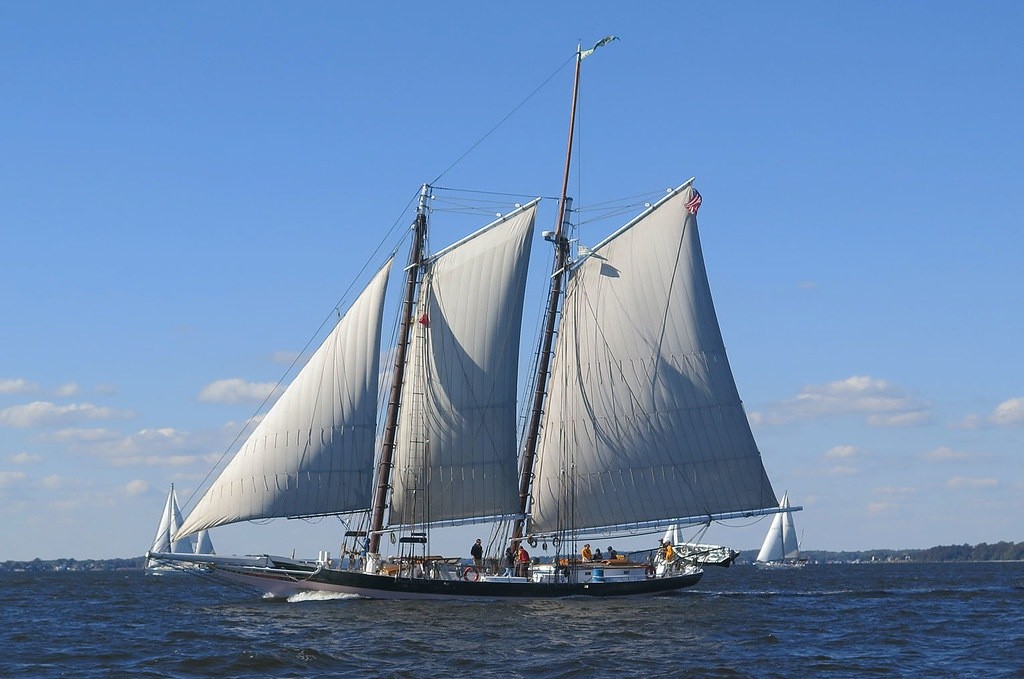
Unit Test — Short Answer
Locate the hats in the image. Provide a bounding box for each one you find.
[475,539,483,543]
[666,540,671,545]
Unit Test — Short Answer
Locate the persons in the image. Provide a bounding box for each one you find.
[607,546,617,559]
[665,540,673,562]
[471,539,483,573]
[593,548,603,559]
[581,544,592,562]
[502,545,529,582]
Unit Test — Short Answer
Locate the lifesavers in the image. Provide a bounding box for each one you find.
[463,567,479,581]
[645,565,656,579]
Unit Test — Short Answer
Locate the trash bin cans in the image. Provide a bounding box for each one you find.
[592,569,603,581]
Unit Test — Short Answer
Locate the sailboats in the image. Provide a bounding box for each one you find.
[144,35,805,597]
[753,490,807,570]
